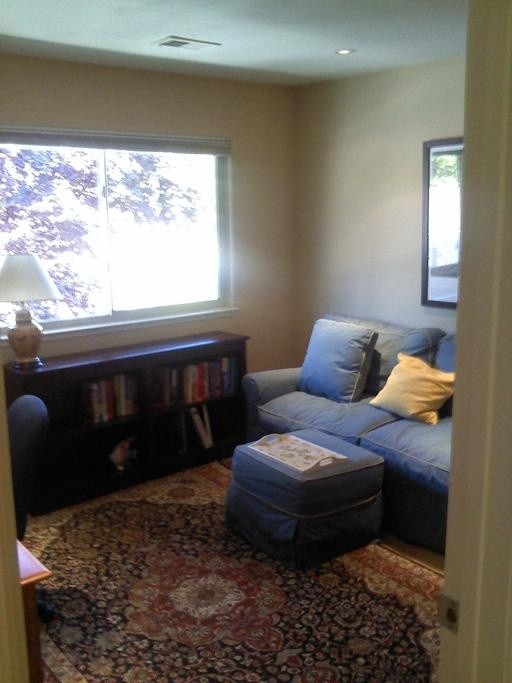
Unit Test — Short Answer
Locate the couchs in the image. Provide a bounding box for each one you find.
[240,314,456,555]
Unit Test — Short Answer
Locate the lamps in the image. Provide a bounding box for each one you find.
[0,253,64,370]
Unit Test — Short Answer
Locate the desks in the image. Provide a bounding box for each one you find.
[17,540,54,683]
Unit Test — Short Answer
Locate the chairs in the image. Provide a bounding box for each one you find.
[7,393,48,540]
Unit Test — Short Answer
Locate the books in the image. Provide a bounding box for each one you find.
[146,347,239,407]
[79,371,139,424]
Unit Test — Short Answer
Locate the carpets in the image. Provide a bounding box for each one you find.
[20,456,446,679]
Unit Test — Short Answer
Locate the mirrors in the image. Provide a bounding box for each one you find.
[421,137,463,308]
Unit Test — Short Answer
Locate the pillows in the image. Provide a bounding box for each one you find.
[299,321,377,403]
[371,352,456,426]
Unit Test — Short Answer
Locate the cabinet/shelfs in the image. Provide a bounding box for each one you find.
[1,331,250,517]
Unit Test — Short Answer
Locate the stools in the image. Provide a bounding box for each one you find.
[226,428,384,567]
